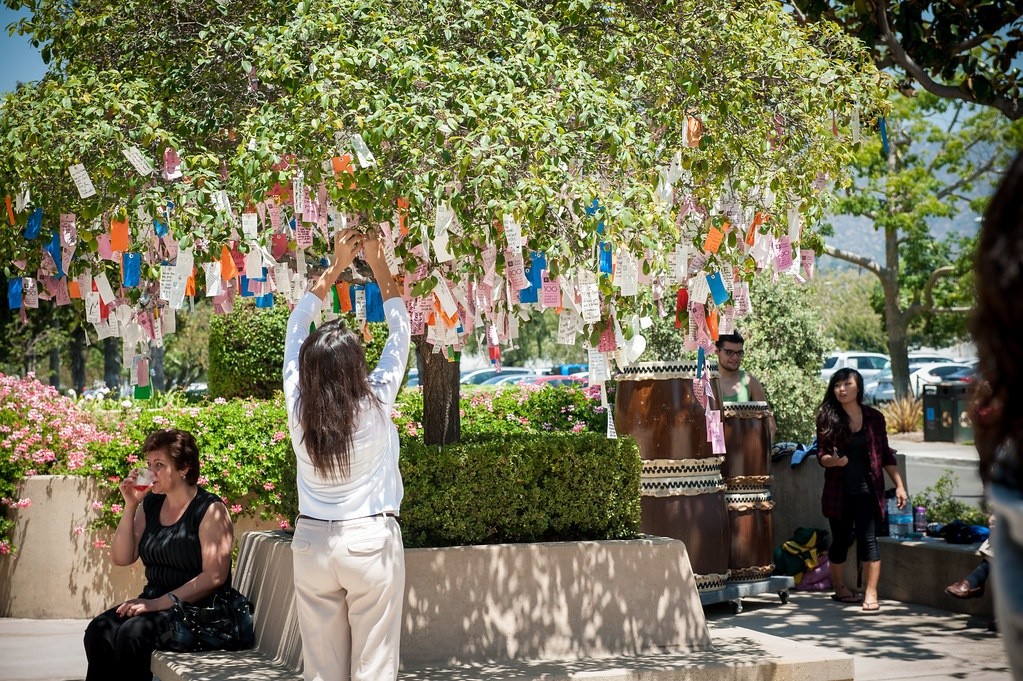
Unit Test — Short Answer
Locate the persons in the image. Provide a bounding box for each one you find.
[945,504,1001,632]
[967,149,1023,681]
[83,429,233,681]
[282,228,411,681]
[817,368,907,610]
[715,330,776,440]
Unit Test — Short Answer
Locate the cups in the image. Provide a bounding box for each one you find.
[131,468,156,492]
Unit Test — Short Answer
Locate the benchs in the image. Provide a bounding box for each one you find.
[147,527,854,681]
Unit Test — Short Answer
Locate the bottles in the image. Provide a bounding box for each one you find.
[886,489,913,541]
[916,507,926,532]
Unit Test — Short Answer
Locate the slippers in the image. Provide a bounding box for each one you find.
[862,600,879,610]
[837,591,863,604]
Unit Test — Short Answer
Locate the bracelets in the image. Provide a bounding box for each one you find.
[314,285,324,300]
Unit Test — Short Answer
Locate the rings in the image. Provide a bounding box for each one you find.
[338,235,343,237]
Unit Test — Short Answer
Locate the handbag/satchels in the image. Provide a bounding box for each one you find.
[159,587,255,653]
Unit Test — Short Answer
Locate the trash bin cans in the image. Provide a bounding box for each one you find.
[922,384,940,442]
[938,382,975,443]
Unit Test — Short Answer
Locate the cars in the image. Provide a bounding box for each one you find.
[863,354,981,404]
[405,364,591,399]
[820,352,889,382]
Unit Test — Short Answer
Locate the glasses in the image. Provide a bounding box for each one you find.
[720,347,744,358]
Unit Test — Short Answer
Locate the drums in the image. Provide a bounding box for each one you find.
[633,474,733,593]
[614,360,727,478]
[722,401,775,494]
[725,491,776,583]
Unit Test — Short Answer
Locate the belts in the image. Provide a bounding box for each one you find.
[299,512,395,522]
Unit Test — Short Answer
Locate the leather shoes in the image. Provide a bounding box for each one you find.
[945,579,985,600]
